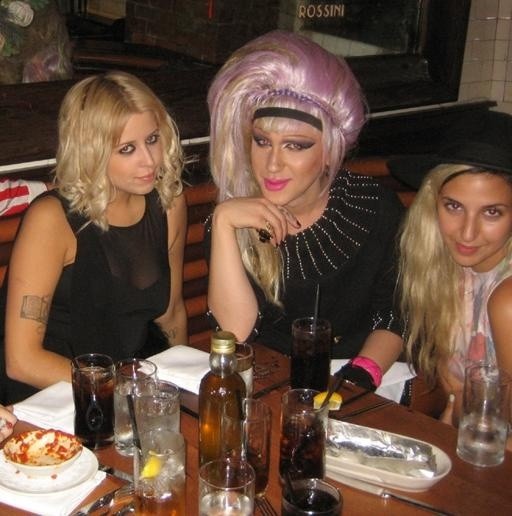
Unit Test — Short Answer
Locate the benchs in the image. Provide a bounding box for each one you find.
[0,157,416,347]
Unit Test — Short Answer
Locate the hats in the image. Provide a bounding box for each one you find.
[386,108,512,188]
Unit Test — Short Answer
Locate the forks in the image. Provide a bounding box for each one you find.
[256,497,279,516]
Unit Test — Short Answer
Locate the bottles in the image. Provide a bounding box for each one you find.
[196,330,248,464]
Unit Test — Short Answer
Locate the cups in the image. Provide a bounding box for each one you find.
[290,317,331,391]
[219,398,272,499]
[71,353,181,457]
[280,477,343,516]
[197,458,256,516]
[278,389,329,487]
[230,342,253,398]
[455,365,512,467]
[133,428,186,516]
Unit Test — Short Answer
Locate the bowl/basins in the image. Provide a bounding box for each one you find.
[2,429,83,476]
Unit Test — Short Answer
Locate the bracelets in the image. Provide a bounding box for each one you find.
[351,356,382,387]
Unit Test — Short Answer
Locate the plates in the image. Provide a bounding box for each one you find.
[0,446,99,494]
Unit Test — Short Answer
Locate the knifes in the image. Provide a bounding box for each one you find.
[326,472,451,515]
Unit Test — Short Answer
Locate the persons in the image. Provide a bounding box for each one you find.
[4,70,187,404]
[398,110,512,453]
[208,30,407,389]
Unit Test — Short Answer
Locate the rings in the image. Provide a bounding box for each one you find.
[266,221,275,239]
